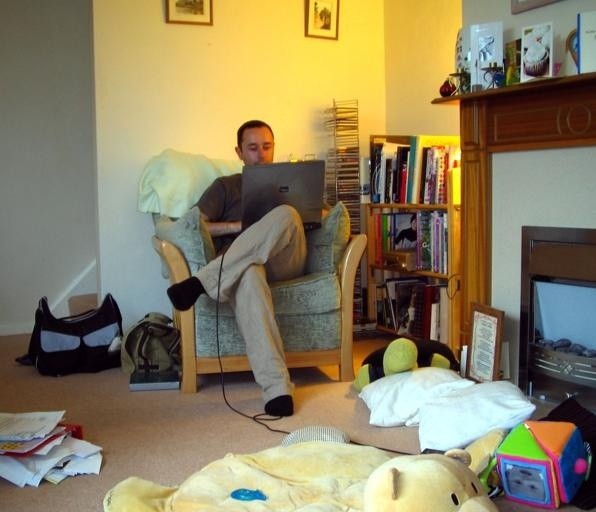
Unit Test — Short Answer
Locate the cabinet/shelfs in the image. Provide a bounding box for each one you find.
[365,202,461,345]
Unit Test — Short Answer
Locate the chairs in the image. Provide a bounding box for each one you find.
[150,161,369,394]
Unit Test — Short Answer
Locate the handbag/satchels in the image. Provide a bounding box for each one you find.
[119,312,180,378]
[33,293,123,377]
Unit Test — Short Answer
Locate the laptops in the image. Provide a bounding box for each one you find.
[240,159,325,235]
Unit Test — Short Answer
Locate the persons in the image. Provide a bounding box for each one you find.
[165,120,331,418]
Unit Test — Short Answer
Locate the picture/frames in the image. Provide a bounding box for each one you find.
[304,0,339,40]
[520,22,554,83]
[469,20,504,92]
[166,0,213,25]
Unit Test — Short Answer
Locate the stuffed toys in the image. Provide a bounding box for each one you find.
[352,336,459,393]
[102,423,499,512]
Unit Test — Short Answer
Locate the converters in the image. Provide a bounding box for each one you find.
[420,449,447,454]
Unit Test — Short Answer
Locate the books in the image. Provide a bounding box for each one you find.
[358,133,460,345]
[0,410,104,489]
